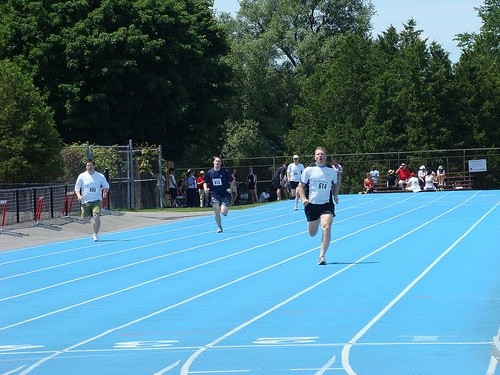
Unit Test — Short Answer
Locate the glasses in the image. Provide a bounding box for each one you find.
[401,165,405,166]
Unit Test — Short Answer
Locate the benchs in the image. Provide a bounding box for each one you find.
[365,171,474,193]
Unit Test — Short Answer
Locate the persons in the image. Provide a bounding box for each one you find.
[74,158,109,242]
[297,145,339,265]
[154,168,212,207]
[286,154,305,210]
[247,167,259,204]
[271,162,296,200]
[229,168,241,206]
[259,187,271,202]
[202,155,234,233]
[330,157,343,204]
[363,162,464,194]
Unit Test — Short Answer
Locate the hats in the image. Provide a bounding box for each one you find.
[200,171,205,175]
[388,169,394,175]
[410,173,415,177]
[419,165,425,170]
[293,155,299,159]
[401,163,406,165]
[438,166,444,169]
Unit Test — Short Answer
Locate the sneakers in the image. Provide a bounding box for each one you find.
[318,256,327,265]
[93,234,98,242]
[295,206,298,210]
[217,228,222,233]
